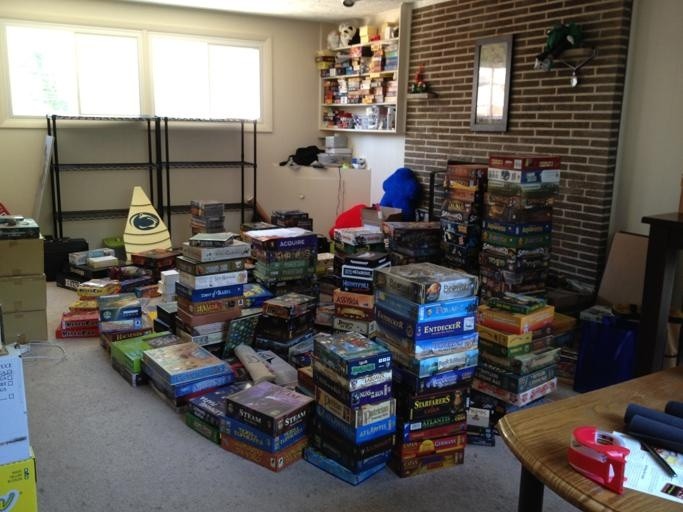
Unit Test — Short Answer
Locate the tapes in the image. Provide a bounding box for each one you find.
[570,430,625,477]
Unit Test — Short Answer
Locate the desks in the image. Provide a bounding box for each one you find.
[637,212,681,376]
[497,363,680,512]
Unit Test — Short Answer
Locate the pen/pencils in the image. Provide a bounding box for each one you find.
[642,442,677,478]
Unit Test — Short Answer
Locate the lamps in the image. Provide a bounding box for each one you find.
[533,24,592,90]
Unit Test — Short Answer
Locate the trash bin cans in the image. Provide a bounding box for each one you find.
[572,309,668,394]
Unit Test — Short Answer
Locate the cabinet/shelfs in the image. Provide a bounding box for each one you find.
[319,39,400,136]
[44,113,259,248]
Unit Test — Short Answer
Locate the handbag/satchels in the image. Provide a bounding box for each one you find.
[573,303,682,394]
[43,234,89,282]
[279,146,326,167]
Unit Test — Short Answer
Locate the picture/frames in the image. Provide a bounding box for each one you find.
[468,36,515,130]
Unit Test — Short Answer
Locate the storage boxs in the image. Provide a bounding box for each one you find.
[0,349,31,461]
[0,231,47,276]
[1,278,47,315]
[0,441,38,512]
[0,312,47,345]
